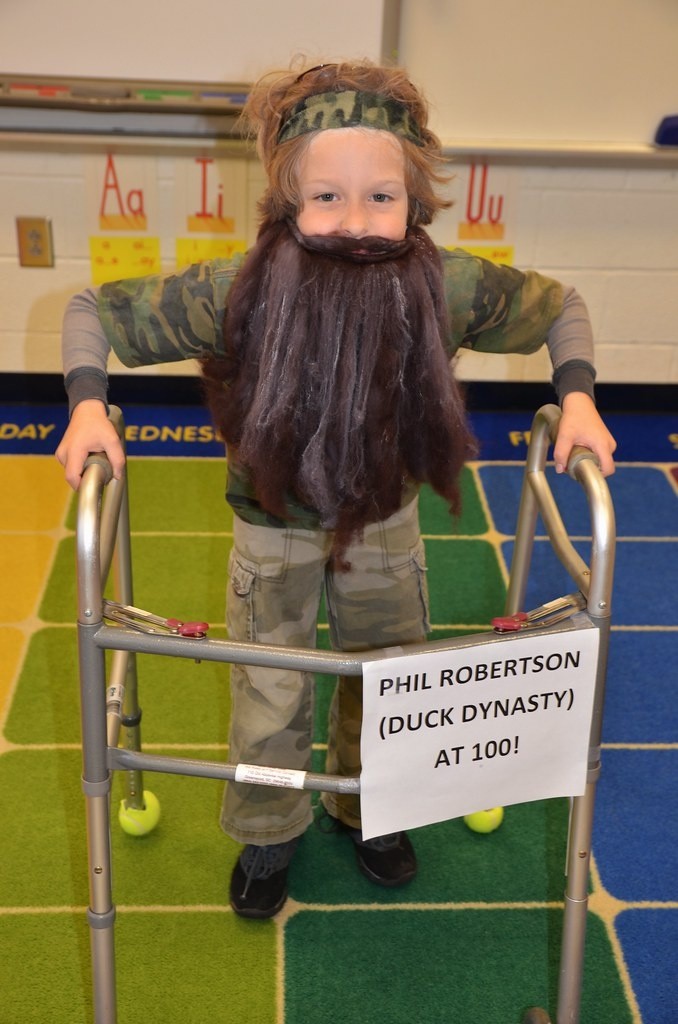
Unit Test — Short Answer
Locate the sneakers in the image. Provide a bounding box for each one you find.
[227,831,303,918]
[323,803,418,887]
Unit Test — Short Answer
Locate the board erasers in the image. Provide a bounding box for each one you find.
[651,113,678,148]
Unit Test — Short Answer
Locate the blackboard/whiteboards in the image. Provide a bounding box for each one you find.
[0,1,678,168]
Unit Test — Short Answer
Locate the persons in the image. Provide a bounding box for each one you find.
[57,61,617,921]
